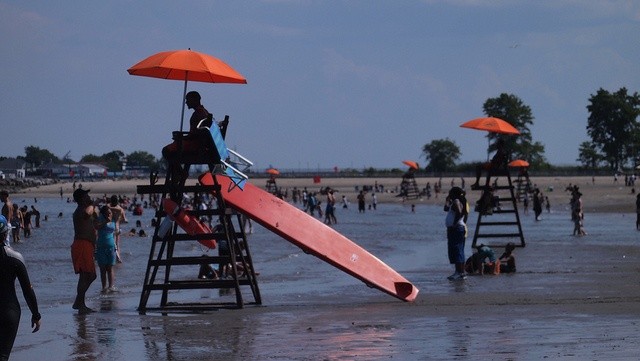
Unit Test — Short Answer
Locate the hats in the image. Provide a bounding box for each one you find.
[73,188,91,201]
[0,215,8,233]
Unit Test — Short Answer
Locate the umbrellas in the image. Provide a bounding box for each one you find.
[459,117,521,163]
[126,48,248,131]
[402,160,418,172]
[508,160,529,179]
[266,169,279,175]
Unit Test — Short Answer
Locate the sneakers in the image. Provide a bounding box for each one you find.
[462,274,467,279]
[450,274,464,280]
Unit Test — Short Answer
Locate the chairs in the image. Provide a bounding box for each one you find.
[172,114,229,186]
[491,141,512,167]
[196,116,254,192]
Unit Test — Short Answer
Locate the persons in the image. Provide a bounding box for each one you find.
[438,177,442,189]
[564,183,588,239]
[129,229,136,236]
[417,183,432,202]
[197,254,222,280]
[623,174,637,186]
[493,178,498,187]
[67,199,70,204]
[434,183,439,199]
[2,215,40,361]
[356,181,400,194]
[278,186,338,225]
[22,205,41,237]
[444,188,470,280]
[95,206,116,294]
[137,229,146,237]
[161,91,210,186]
[451,178,455,188]
[57,213,64,218]
[12,204,25,243]
[70,189,102,315]
[97,194,161,217]
[532,191,544,221]
[460,177,465,190]
[472,136,517,188]
[357,192,366,214]
[523,194,529,215]
[212,215,230,280]
[180,193,219,231]
[410,205,416,212]
[340,196,349,209]
[72,182,75,189]
[545,197,551,213]
[464,244,495,276]
[371,193,376,209]
[532,184,540,197]
[635,194,640,232]
[42,214,50,222]
[613,174,618,183]
[34,197,37,203]
[59,187,64,199]
[498,242,516,273]
[0,191,13,244]
[107,195,128,263]
[367,205,372,210]
[243,215,252,234]
[135,221,142,228]
